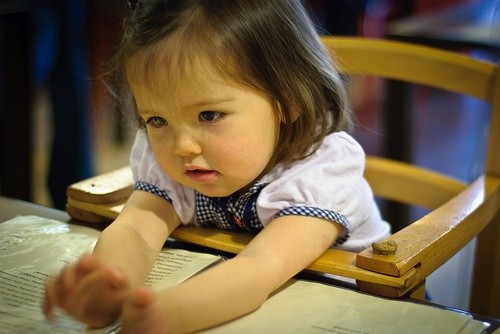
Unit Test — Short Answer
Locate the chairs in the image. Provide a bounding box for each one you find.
[67,35,500,302]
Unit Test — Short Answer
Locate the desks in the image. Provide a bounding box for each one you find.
[0,198,500,334]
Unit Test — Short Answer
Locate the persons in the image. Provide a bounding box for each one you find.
[0,0,96,213]
[42,0,393,334]
